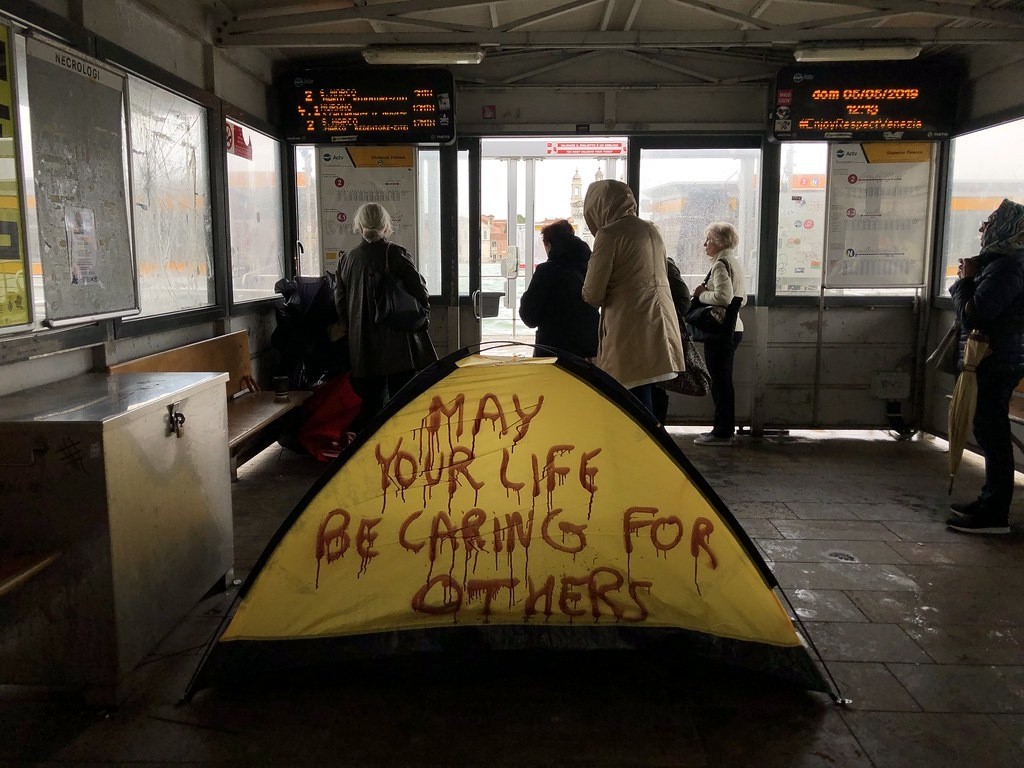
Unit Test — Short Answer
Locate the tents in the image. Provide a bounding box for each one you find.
[175,338,856,710]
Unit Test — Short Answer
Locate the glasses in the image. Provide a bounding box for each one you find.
[982,221,991,228]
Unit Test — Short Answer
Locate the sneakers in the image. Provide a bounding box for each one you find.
[946,504,1010,533]
[950,499,981,517]
[694,432,736,445]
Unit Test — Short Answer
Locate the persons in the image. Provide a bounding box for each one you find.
[582,179,684,421]
[947,199,1024,536]
[335,203,441,411]
[692,221,748,446]
[519,218,600,363]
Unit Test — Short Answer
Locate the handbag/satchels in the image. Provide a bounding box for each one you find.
[369,268,430,333]
[926,323,961,375]
[654,313,711,396]
[686,292,743,342]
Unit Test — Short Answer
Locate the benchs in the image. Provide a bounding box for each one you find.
[106,328,318,489]
[948,370,1023,463]
